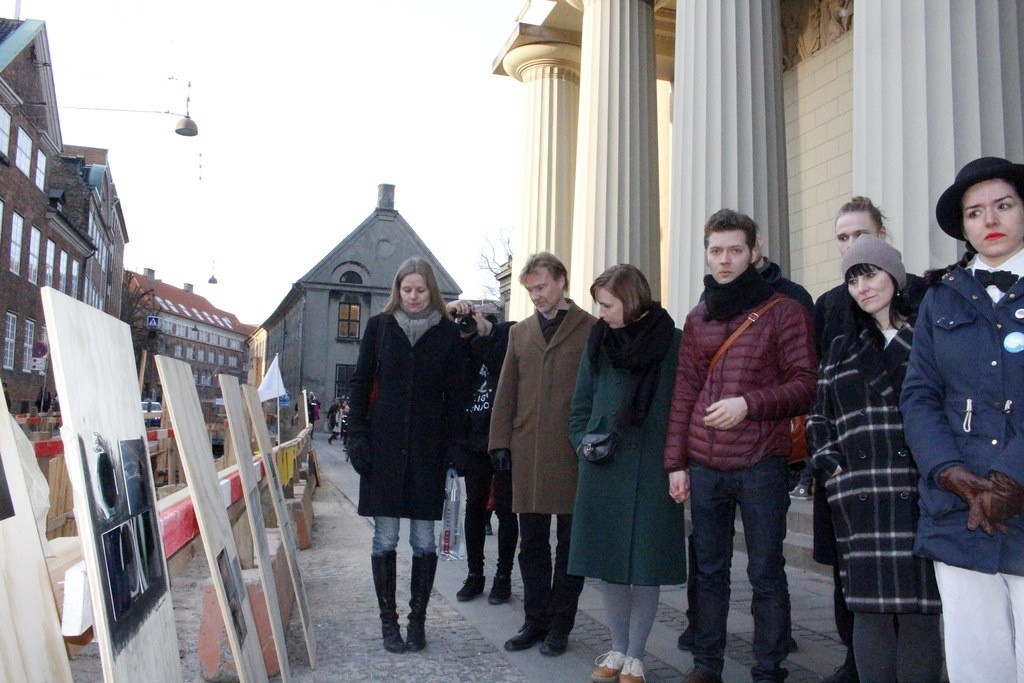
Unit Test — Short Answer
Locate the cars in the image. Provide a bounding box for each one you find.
[142,401,163,426]
[212,397,224,412]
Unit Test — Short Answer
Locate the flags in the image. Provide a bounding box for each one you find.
[257,355,286,402]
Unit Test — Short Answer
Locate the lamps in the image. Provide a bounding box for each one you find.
[174,81,198,136]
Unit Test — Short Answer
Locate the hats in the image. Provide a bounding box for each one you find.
[843,234,906,291]
[936,157,1024,241]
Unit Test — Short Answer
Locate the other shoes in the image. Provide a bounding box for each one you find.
[681,669,722,683]
[678,624,693,650]
[789,638,797,654]
[821,647,860,683]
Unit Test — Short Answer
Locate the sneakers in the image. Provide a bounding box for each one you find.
[620,657,647,683]
[457,576,485,601]
[591,651,626,683]
[487,577,511,604]
[789,484,810,500]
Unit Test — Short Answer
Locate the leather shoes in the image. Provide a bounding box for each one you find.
[540,628,568,654]
[504,623,545,651]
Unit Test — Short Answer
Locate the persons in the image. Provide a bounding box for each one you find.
[565,263,687,683]
[35,386,51,413]
[326,399,351,444]
[664,196,941,683]
[3,383,11,410]
[898,156,1024,683]
[346,257,463,653]
[442,296,519,607]
[485,252,598,657]
[307,392,320,439]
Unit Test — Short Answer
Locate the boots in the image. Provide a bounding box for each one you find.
[372,552,405,653]
[328,436,333,444]
[485,509,493,534]
[405,556,439,651]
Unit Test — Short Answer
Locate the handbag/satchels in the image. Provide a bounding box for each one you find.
[368,379,379,411]
[314,403,319,421]
[790,414,810,465]
[439,467,461,561]
[576,431,620,465]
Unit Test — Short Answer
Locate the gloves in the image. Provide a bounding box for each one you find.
[490,448,513,475]
[940,464,1011,537]
[968,473,1023,530]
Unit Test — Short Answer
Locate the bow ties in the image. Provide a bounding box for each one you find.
[974,268,1019,292]
